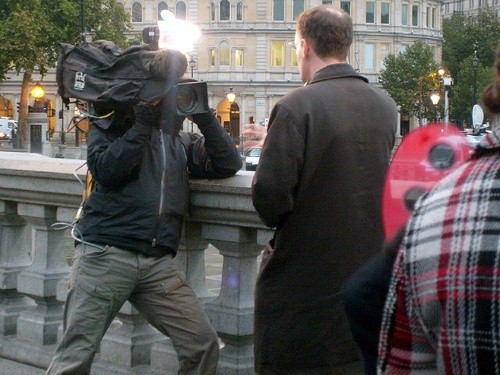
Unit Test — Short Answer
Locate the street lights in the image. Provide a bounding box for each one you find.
[472,50,480,105]
[189,56,196,132]
[227,86,244,156]
[431,80,442,124]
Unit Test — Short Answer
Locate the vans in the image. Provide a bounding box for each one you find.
[0,119,18,138]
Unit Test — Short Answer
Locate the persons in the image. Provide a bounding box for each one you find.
[378,44,500,374]
[252,6,398,374]
[46,42,243,375]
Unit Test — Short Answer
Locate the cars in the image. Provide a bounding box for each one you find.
[0,133,10,139]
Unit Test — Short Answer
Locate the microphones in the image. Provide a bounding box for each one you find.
[148,50,188,80]
